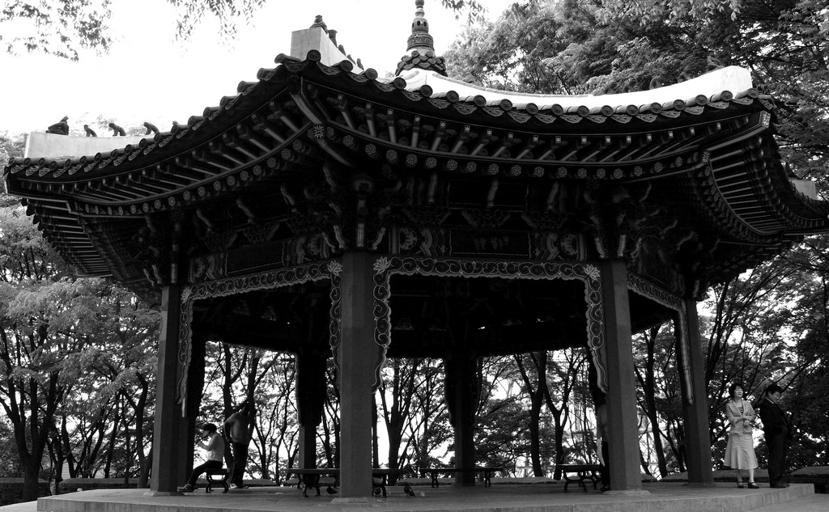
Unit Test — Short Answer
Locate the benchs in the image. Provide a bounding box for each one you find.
[288,464,503,496]
[559,461,602,491]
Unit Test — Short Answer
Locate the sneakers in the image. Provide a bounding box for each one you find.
[230,483,249,489]
[177,483,196,492]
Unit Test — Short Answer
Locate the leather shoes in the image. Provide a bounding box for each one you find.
[748,481,760,489]
[737,480,747,489]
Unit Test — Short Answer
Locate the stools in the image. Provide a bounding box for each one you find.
[206,466,230,493]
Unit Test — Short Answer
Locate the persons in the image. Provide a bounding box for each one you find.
[176,424,225,493]
[723,383,759,488]
[595,402,610,492]
[760,385,792,488]
[222,402,256,489]
